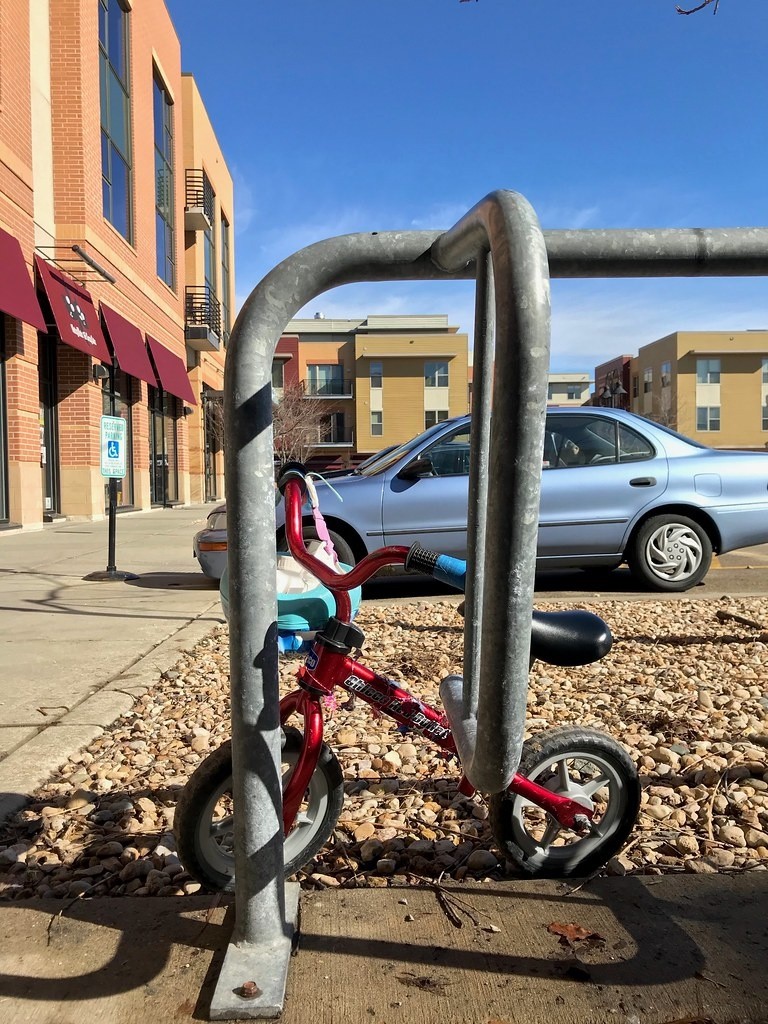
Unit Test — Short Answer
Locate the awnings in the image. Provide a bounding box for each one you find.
[146,333,198,406]
[34,252,112,365]
[0,228,48,334]
[99,300,158,388]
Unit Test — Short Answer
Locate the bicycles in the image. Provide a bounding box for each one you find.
[174,461,643,879]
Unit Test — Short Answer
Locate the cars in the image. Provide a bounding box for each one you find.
[192,405,768,594]
